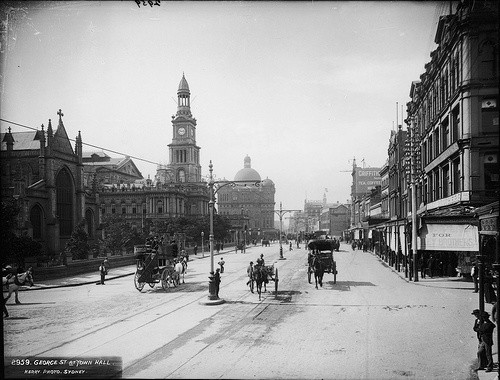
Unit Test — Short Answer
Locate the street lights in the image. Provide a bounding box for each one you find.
[272,204,301,258]
[207,159,264,300]
[200,231,204,258]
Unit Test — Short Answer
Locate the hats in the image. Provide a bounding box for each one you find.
[471,310,479,314]
[479,312,491,316]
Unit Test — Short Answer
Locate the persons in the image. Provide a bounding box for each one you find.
[476,312,494,372]
[99,262,107,285]
[471,309,484,370]
[216,241,223,252]
[104,257,110,275]
[247,262,254,288]
[194,244,198,255]
[237,231,317,251]
[325,236,340,251]
[3,296,9,318]
[352,240,368,252]
[470,262,479,293]
[257,254,264,265]
[218,258,225,273]
[145,235,178,258]
[427,254,435,277]
[418,254,427,278]
[1,260,34,290]
[310,244,320,256]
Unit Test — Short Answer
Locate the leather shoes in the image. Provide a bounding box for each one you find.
[476,367,484,370]
[485,368,492,372]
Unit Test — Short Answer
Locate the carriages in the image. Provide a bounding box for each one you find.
[134,250,189,292]
[247,264,279,301]
[307,239,337,289]
[235,241,246,254]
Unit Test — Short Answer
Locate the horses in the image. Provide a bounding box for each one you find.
[253,265,269,301]
[312,253,326,290]
[2,266,35,304]
[174,255,190,285]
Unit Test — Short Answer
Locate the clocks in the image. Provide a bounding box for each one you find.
[178,127,186,136]
